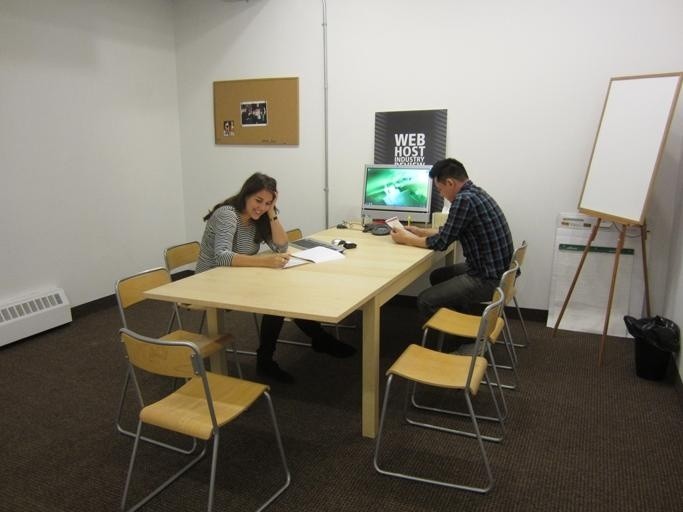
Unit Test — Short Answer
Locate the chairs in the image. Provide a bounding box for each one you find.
[115,328,292,512]
[162,240,238,336]
[251,228,358,350]
[410,259,519,422]
[495,240,530,353]
[114,267,244,455]
[369,287,507,494]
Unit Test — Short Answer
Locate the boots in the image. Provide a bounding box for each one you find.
[256,346,294,384]
[297,319,357,359]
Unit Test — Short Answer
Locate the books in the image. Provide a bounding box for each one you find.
[384,216,403,233]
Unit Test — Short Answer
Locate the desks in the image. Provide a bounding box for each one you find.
[141,210,459,439]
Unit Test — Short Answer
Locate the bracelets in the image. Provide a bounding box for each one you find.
[269,216,277,222]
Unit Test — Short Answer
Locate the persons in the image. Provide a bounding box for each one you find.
[241,104,259,124]
[392,159,514,353]
[256,106,267,124]
[230,120,235,136]
[196,173,357,386]
[224,121,231,135]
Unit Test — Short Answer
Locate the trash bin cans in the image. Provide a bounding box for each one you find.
[623,312,683,384]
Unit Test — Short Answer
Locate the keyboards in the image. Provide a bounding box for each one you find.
[288,236,347,253]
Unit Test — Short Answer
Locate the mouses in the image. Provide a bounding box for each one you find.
[344,240,357,248]
[332,238,345,245]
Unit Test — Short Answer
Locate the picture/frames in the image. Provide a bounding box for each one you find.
[221,120,236,137]
[239,99,269,128]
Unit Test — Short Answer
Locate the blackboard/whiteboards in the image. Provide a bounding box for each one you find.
[577,70,683,226]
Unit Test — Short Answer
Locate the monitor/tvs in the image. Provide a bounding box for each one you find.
[360,163,434,224]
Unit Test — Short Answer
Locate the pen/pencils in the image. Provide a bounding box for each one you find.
[268,240,281,254]
[407,215,412,226]
